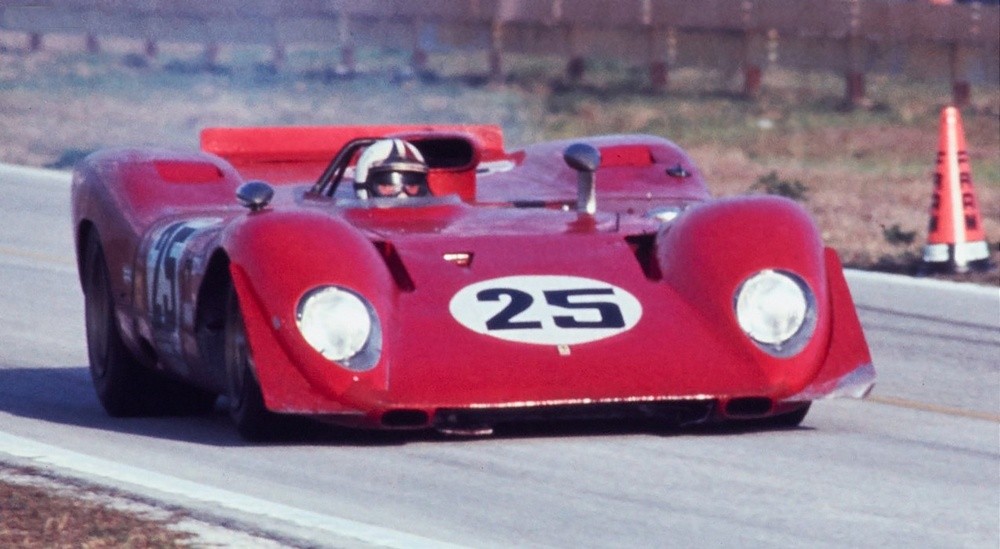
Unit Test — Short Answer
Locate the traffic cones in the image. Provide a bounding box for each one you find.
[906,104,996,284]
[734,30,773,108]
[641,25,678,96]
[840,57,878,115]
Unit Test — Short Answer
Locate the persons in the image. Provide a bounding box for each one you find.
[350,140,435,202]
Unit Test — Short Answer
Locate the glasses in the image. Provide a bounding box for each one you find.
[369,169,428,197]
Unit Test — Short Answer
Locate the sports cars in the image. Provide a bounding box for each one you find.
[70,123,878,446]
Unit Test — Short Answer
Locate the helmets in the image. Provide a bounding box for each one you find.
[354,140,428,202]
